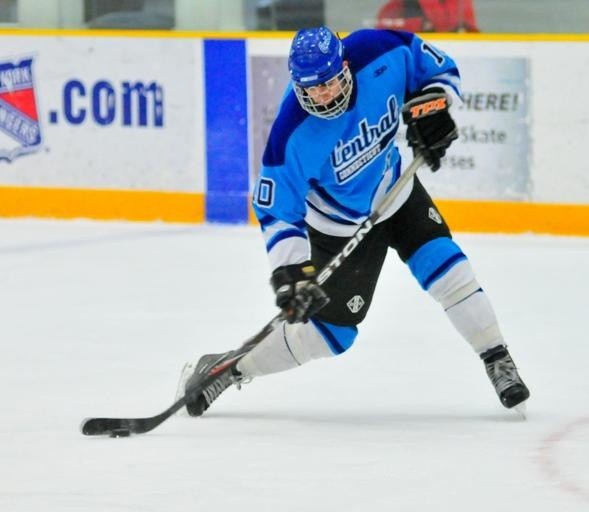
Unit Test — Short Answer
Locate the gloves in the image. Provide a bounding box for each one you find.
[402,87,458,172]
[273,260,330,324]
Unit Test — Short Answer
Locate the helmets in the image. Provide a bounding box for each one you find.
[288,24,352,120]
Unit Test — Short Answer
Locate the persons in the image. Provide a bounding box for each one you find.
[185,26,530,416]
[375,0,481,32]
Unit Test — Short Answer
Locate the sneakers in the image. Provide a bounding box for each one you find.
[186,343,256,416]
[480,345,528,408]
